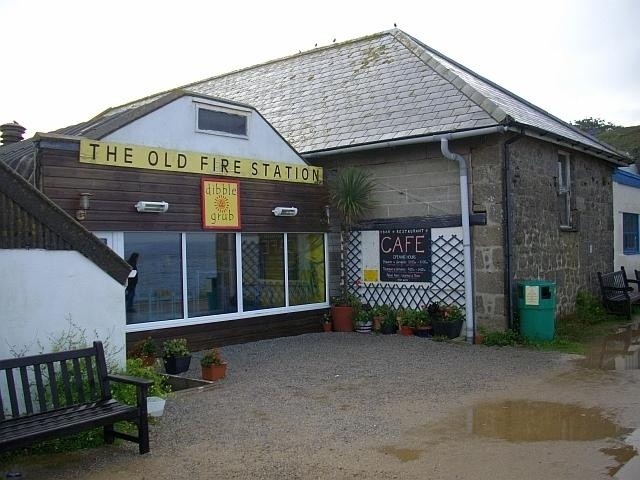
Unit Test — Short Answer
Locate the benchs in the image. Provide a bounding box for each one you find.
[0,339,156,460]
[597,264,640,321]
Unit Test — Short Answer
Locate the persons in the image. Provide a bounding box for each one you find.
[125,253,140,313]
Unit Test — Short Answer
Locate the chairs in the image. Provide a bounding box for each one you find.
[133,275,214,320]
[634,269,640,293]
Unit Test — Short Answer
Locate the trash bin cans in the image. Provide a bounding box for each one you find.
[517,280,557,341]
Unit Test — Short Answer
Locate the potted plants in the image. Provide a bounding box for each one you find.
[160,336,194,375]
[199,344,227,381]
[319,290,464,340]
[323,165,380,333]
[133,357,177,419]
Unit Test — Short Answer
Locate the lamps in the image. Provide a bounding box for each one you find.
[74,190,97,221]
[319,202,333,228]
[270,207,299,217]
[132,200,169,213]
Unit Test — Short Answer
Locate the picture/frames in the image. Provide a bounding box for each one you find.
[201,175,242,230]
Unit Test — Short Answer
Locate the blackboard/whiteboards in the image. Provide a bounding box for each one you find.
[379,228,432,282]
